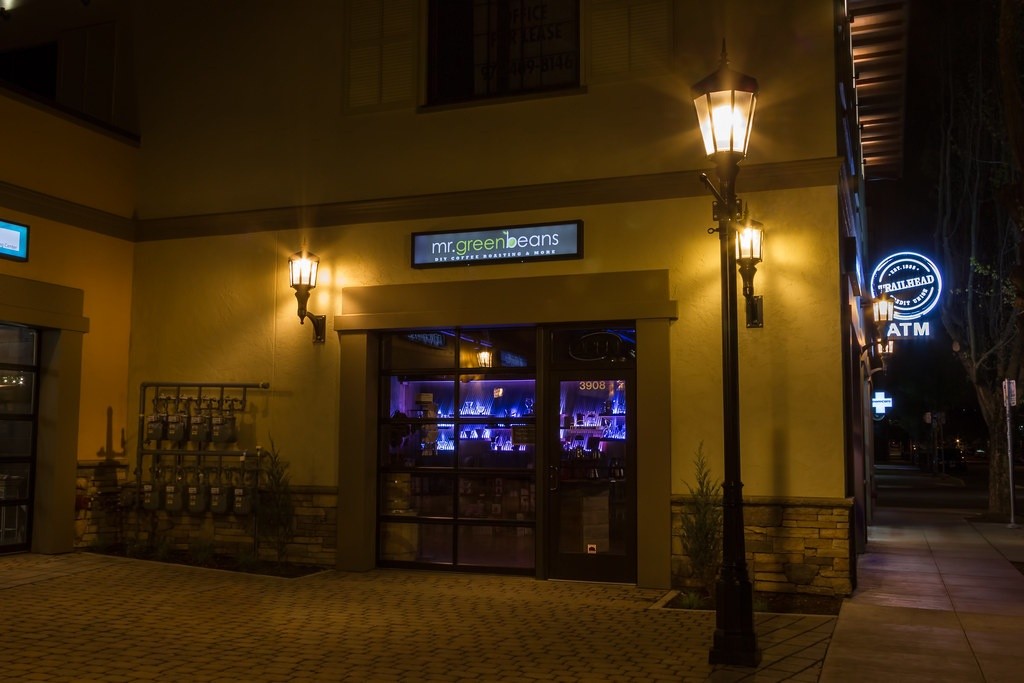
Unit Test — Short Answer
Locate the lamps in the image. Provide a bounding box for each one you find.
[861,338,896,381]
[733,201,765,329]
[286,235,326,345]
[856,287,895,356]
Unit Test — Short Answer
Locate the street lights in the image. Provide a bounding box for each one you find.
[690,36,764,666]
